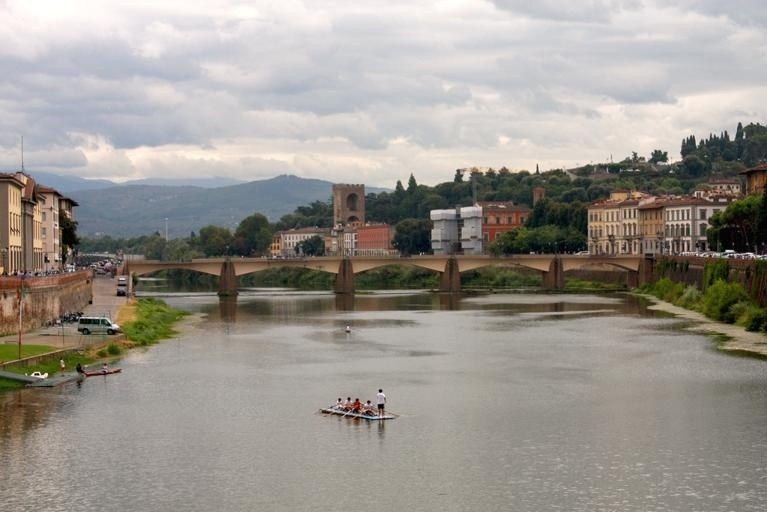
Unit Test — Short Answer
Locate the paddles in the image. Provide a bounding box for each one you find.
[342,409,354,418]
[385,411,401,418]
[323,407,341,418]
[314,404,337,415]
[351,411,362,421]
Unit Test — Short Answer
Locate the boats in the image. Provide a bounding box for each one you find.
[80,367,122,377]
[320,403,395,419]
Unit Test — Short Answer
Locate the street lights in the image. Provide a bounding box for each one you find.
[746,242,748,260]
[733,243,734,258]
[720,243,721,257]
[762,242,764,260]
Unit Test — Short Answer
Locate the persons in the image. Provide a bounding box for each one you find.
[362,400,377,416]
[351,398,364,414]
[75,362,86,373]
[59,356,66,377]
[374,388,387,418]
[344,396,353,412]
[101,362,109,374]
[336,397,344,411]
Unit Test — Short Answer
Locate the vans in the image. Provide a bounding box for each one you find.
[78,317,119,335]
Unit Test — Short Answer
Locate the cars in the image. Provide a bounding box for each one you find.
[574,251,591,256]
[117,277,126,296]
[679,250,767,259]
[90,260,112,275]
